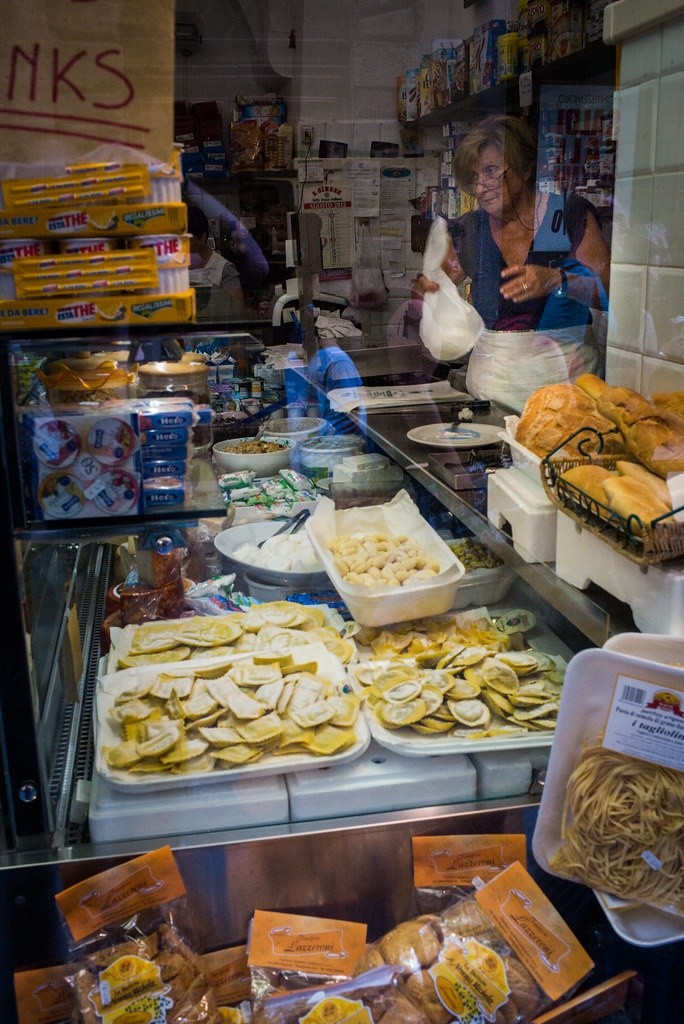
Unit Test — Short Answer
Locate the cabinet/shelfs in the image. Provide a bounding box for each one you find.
[4,323,232,530]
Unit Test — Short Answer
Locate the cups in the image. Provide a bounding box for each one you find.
[243,571,316,600]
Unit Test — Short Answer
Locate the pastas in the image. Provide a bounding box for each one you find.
[547,731,684,913]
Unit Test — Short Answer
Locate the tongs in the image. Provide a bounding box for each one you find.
[256,509,311,550]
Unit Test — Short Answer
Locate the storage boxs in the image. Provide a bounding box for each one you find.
[555,508,684,637]
[0,0,185,238]
[484,467,561,563]
[0,234,196,329]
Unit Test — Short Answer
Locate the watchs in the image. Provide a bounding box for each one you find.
[552,267,569,300]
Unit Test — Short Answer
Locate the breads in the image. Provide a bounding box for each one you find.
[71,901,549,1024]
[513,373,684,540]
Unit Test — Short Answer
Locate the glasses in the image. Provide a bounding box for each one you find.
[461,165,511,195]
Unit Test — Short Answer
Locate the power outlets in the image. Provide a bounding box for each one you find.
[301,125,314,145]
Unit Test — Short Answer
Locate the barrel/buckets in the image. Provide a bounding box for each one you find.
[260,417,327,472]
[294,436,364,488]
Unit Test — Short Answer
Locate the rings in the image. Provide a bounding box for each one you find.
[522,281,529,292]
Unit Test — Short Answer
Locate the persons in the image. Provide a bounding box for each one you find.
[184,205,243,317]
[284,217,475,530]
[411,116,612,418]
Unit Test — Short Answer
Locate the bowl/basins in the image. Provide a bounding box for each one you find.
[212,437,297,475]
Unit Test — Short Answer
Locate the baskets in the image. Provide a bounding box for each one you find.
[539,452,684,567]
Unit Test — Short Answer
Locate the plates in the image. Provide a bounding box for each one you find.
[213,521,330,586]
[407,422,508,448]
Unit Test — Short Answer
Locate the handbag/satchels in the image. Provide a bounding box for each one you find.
[420,217,483,360]
